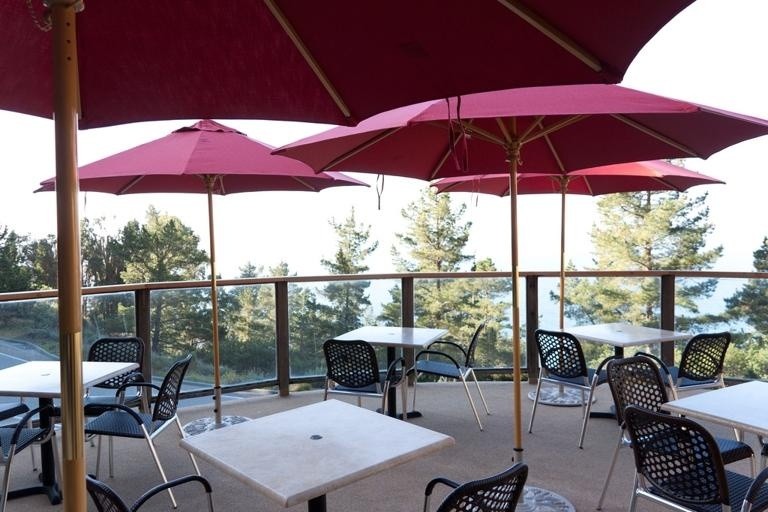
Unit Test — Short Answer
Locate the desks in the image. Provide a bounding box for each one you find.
[557,321,691,420]
[177,397,457,511]
[659,379,767,439]
[0,360,140,505]
[332,325,450,420]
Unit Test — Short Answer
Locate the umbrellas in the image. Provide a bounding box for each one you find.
[269,83,768,512]
[432,159,725,405]
[0,0,694,511]
[32,119,371,440]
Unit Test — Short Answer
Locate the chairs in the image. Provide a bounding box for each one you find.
[0,403,62,512]
[527,328,626,448]
[632,331,741,442]
[83,353,202,508]
[596,356,756,511]
[422,461,529,511]
[81,336,156,478]
[322,338,404,415]
[622,404,767,512]
[405,320,491,432]
[84,472,215,511]
